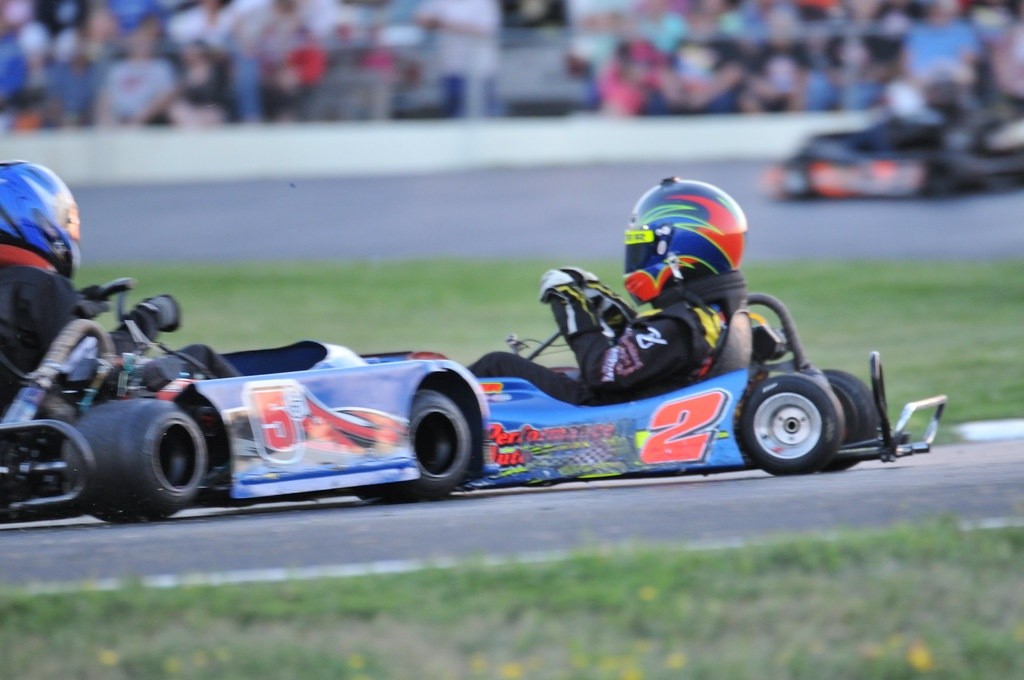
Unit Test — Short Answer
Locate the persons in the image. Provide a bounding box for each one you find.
[0,155,96,418]
[2,1,506,135]
[556,0,1023,118]
[905,65,999,144]
[538,172,756,398]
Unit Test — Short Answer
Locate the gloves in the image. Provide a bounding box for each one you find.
[561,266,601,292]
[140,294,179,332]
[539,269,576,302]
[74,286,109,318]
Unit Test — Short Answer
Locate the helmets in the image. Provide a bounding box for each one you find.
[924,63,977,113]
[621,176,746,306]
[0,159,80,279]
